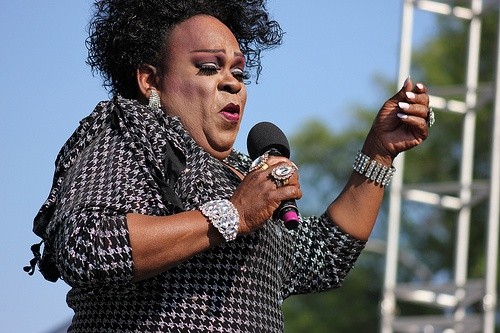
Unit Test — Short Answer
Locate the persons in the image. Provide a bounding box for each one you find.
[22,0,436,333]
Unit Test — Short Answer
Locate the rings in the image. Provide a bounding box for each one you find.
[427,108,435,127]
[249,154,269,173]
[272,163,295,188]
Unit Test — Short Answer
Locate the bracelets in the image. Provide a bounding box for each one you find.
[199,199,240,243]
[352,151,396,186]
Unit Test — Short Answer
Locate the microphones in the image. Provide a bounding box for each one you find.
[247,122,299,230]
